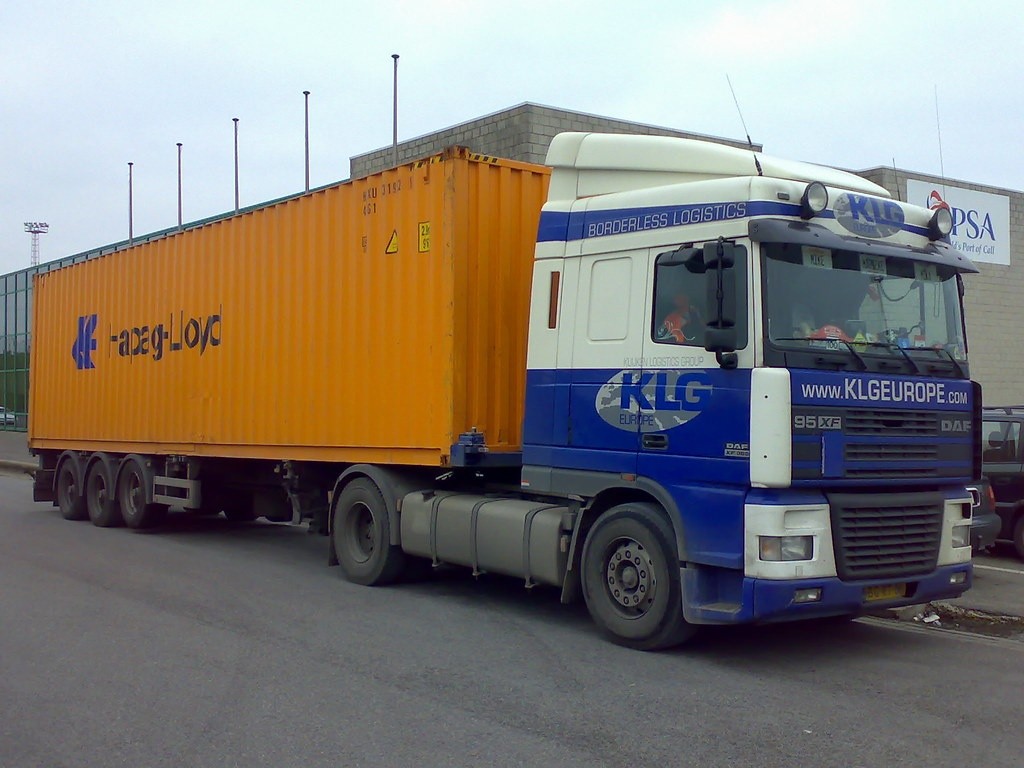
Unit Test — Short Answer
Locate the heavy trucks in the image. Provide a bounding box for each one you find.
[27,130,984,652]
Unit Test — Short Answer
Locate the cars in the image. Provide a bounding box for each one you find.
[0,407,14,424]
[970,406,1024,561]
